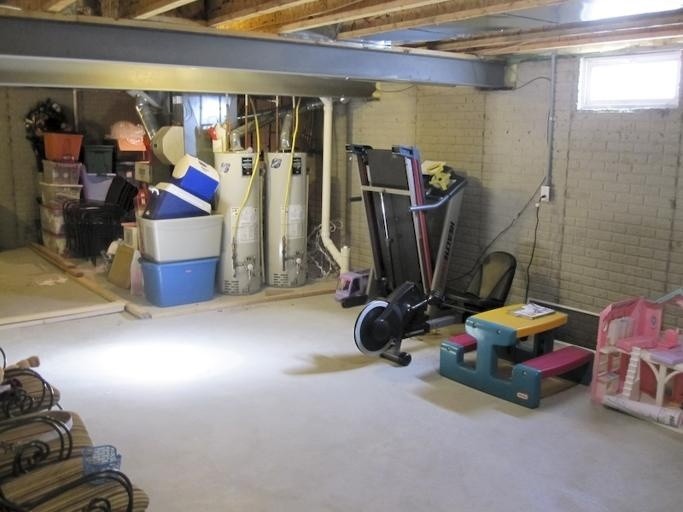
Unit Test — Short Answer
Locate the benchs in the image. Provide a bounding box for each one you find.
[439,334,477,382]
[511,346,594,409]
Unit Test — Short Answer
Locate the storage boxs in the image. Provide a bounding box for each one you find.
[39,132,117,254]
[123,225,139,249]
[137,258,219,307]
[172,153,220,202]
[134,160,171,183]
[143,181,212,219]
[137,212,224,263]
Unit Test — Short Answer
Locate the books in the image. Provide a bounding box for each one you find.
[508,302,555,320]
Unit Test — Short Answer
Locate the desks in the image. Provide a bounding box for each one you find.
[464,304,568,395]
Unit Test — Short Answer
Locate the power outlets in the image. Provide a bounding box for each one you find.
[540,186,550,202]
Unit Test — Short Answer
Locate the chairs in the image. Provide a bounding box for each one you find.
[0,347,149,511]
